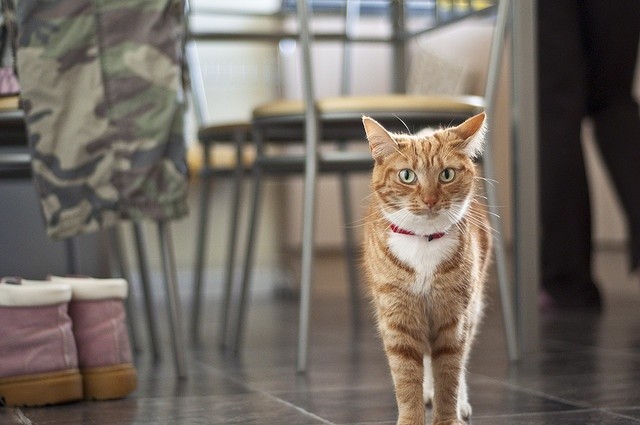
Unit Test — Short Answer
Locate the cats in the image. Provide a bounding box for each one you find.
[341,109,508,425]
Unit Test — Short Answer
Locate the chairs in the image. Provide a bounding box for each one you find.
[186,0,376,355]
[232,0,517,373]
[1,0,188,383]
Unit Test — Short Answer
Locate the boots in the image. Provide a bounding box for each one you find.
[2,278,85,410]
[51,276,138,402]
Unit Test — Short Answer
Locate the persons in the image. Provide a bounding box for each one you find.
[537,45,640,350]
